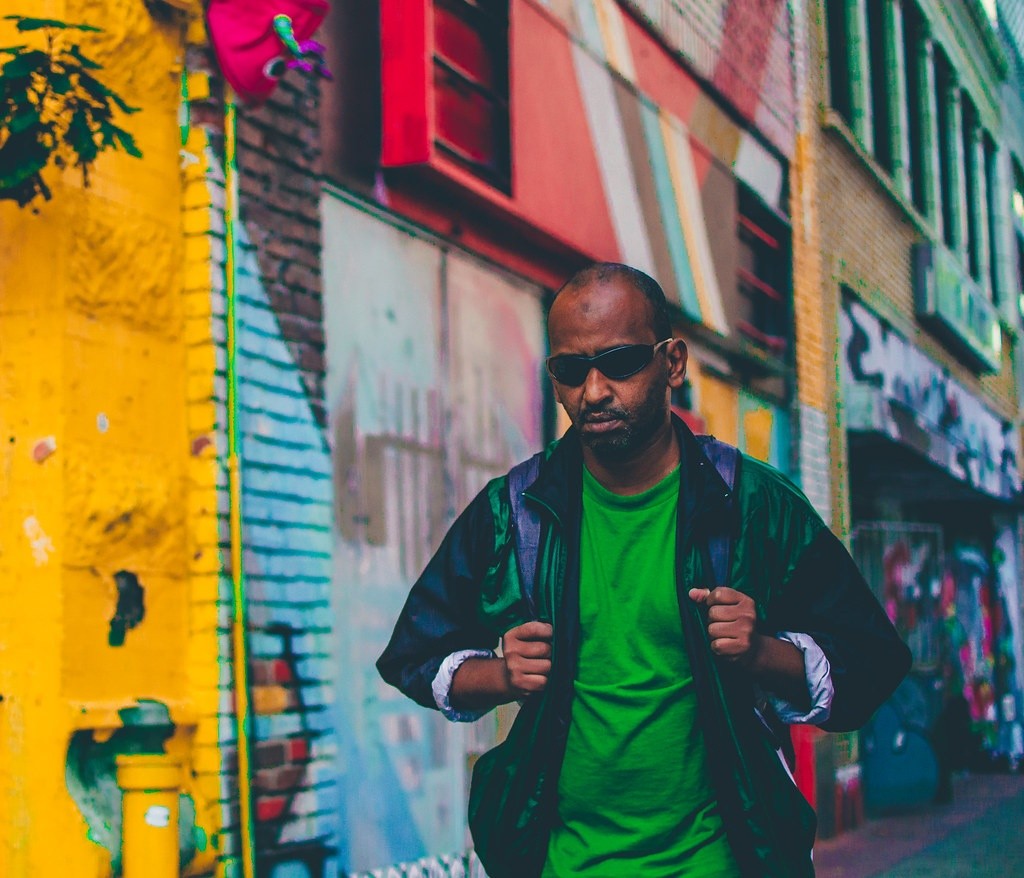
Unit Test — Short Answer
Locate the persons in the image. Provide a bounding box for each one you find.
[374,261,913,878]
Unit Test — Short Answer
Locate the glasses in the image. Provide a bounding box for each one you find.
[548,339,673,387]
